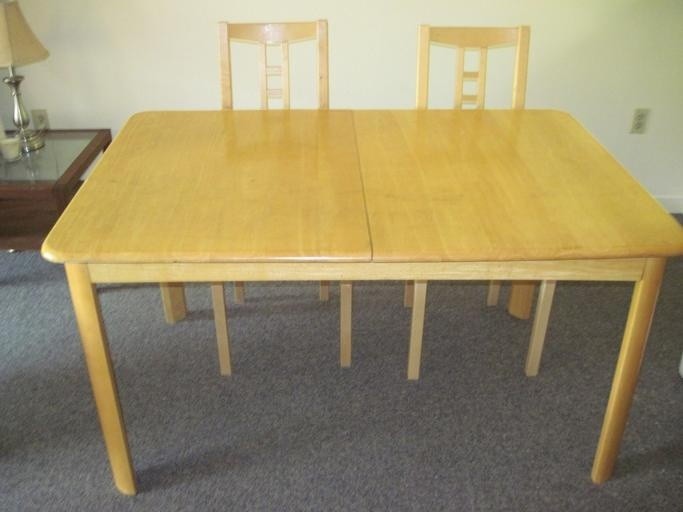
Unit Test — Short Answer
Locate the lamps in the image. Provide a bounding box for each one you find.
[0,0,49,153]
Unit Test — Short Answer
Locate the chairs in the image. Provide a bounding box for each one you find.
[210,20,352,377]
[404,24,559,380]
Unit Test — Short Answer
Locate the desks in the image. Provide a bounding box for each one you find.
[0,130,112,254]
[41,110,683,496]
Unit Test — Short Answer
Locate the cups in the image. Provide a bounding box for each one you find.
[0,130,22,161]
